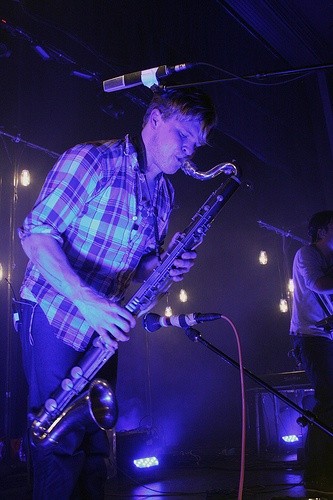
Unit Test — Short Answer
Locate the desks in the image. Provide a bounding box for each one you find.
[246,384,315,456]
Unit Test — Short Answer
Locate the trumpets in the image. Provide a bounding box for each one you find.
[31,163,243,446]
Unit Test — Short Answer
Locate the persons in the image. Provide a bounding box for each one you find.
[290,211,333,495]
[18,84,217,500]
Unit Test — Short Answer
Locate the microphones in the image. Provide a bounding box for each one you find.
[142,312,222,333]
[103,62,192,93]
